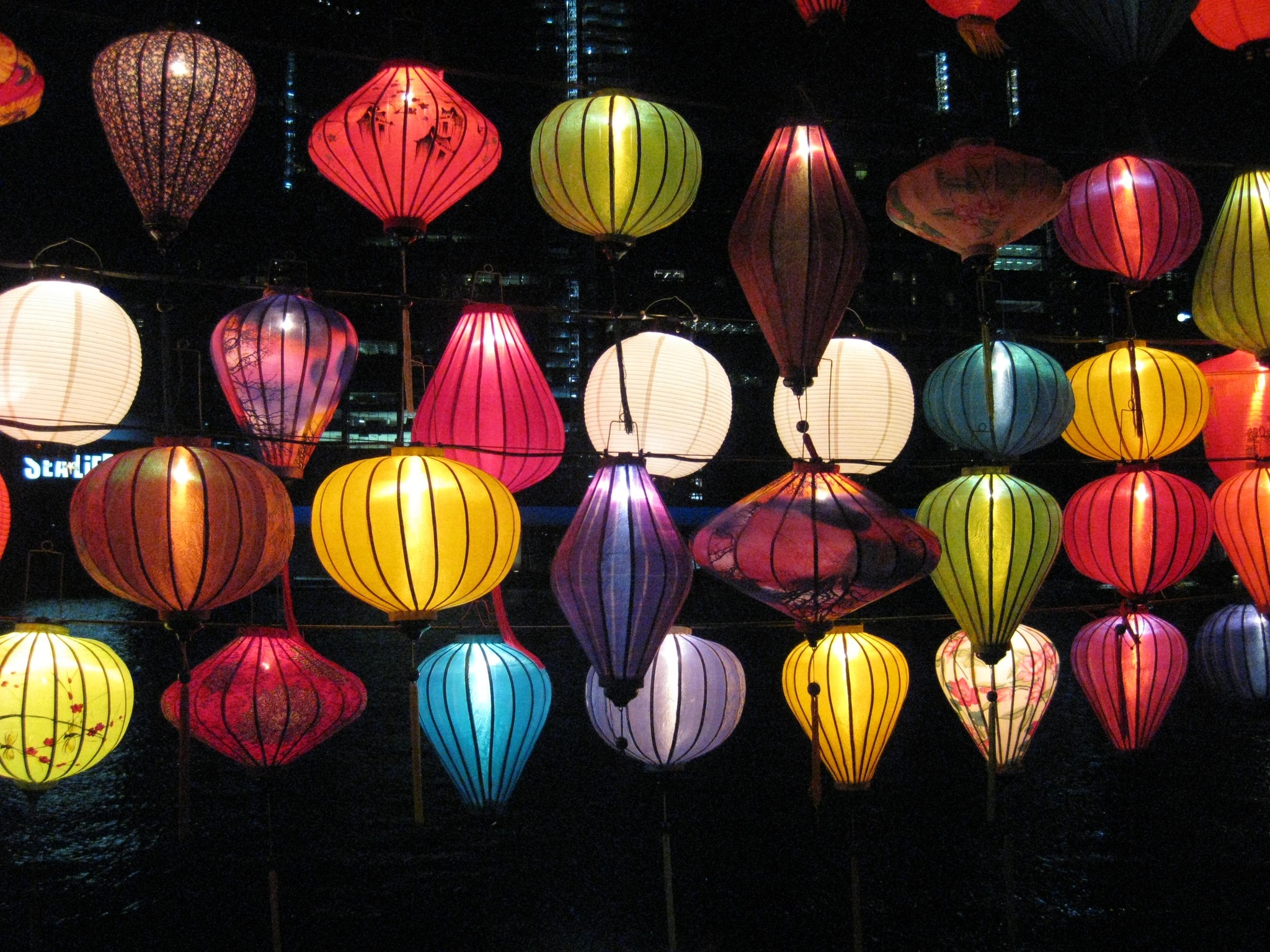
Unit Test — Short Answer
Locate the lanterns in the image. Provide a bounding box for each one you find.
[0,0,1270,818]
